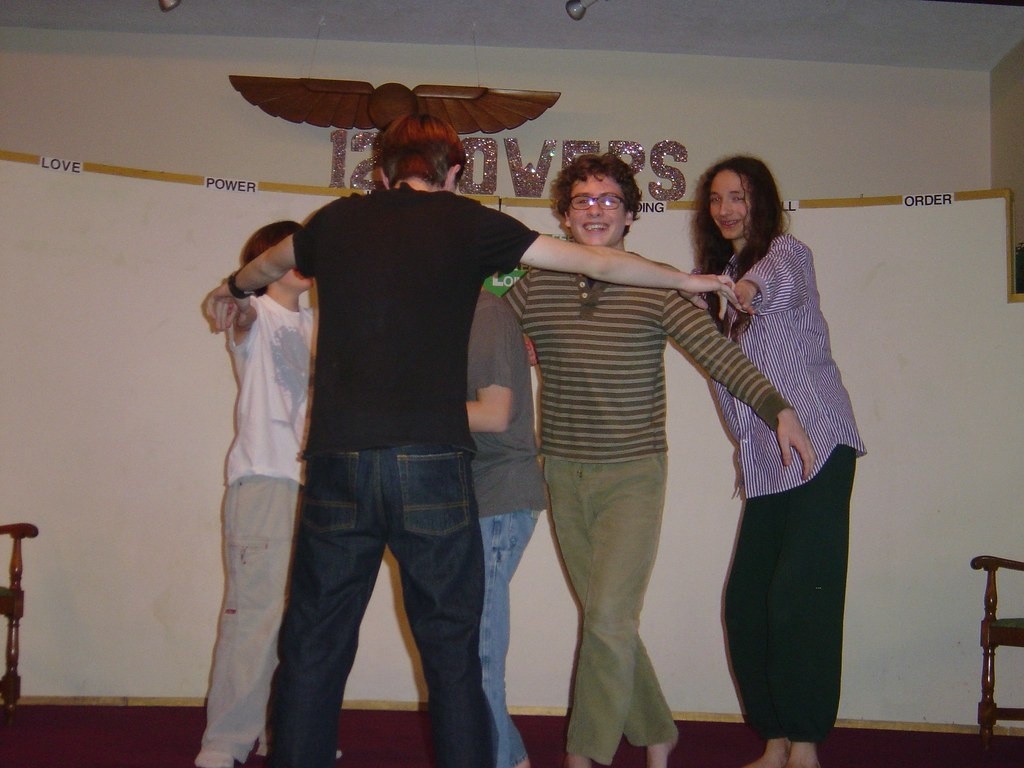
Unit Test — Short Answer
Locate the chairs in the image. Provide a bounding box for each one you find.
[0,522,38,726]
[969,554,1023,749]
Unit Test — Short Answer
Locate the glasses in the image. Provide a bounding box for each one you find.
[569,195,626,210]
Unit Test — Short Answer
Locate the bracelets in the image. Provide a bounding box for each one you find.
[229,274,252,298]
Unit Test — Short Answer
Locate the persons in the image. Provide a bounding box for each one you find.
[500,153,868,768]
[195,112,748,768]
